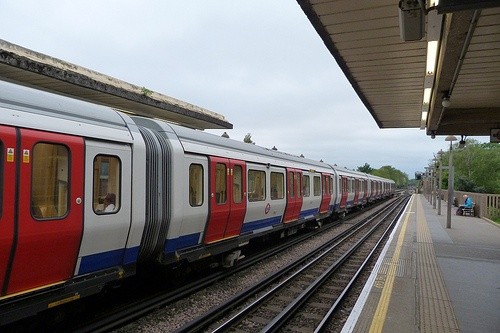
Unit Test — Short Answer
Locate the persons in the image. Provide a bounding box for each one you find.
[456,195,472,216]
[271,181,278,200]
[95,193,116,212]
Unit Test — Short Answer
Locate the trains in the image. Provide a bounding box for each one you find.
[0,79,397,329]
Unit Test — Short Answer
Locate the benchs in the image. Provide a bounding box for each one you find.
[463,202,477,217]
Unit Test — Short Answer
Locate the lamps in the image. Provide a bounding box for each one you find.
[442,96,451,108]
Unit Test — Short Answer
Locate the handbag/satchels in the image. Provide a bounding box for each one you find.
[456,209,463,215]
